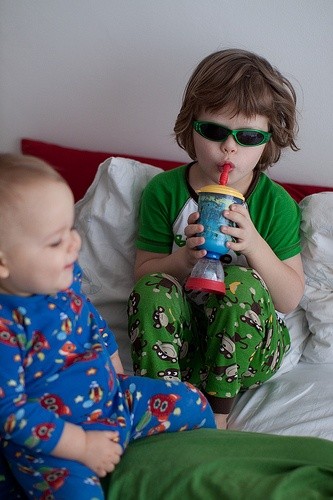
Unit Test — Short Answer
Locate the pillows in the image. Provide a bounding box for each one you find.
[70,156,310,382]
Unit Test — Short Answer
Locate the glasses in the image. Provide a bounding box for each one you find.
[192,120,272,146]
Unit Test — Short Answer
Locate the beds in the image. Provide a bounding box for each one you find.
[20,137,333,444]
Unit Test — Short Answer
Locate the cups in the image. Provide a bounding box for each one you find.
[185,185,245,295]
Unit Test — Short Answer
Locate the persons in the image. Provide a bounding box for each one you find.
[0,151,219,500]
[127,50,304,431]
